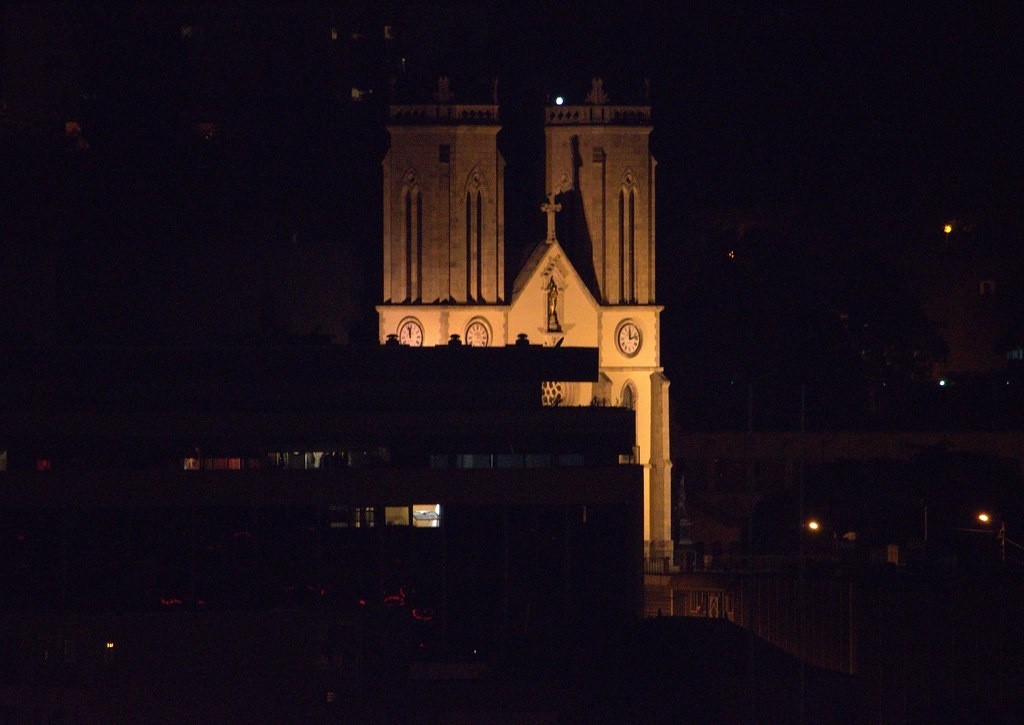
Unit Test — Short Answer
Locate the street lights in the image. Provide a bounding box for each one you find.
[977,514,1006,719]
[809,520,856,677]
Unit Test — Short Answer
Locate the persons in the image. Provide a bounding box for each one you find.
[548,286,558,315]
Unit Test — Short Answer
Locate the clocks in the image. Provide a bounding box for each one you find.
[614,318,645,358]
[397,316,425,347]
[461,314,493,347]
